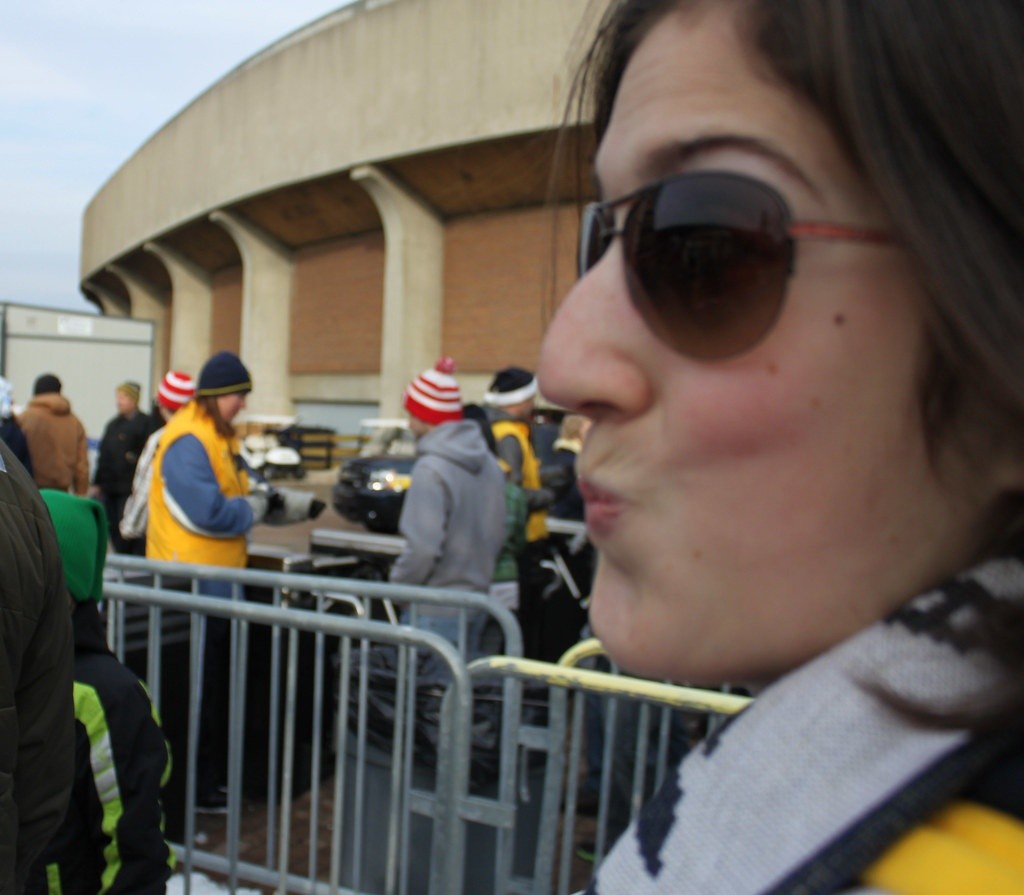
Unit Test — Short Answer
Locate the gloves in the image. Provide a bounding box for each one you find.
[309,500,326,518]
[264,493,284,515]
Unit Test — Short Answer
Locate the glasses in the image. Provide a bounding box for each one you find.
[575,169,904,363]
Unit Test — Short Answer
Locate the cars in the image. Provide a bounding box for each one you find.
[330,454,425,534]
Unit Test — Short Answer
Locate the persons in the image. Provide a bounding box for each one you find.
[385,356,743,865]
[22,487,176,895]
[0,431,76,895]
[16,375,89,497]
[0,376,36,482]
[89,352,326,847]
[538,0,1024,894]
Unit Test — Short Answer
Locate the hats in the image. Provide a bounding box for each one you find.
[404,356,463,424]
[198,352,253,395]
[486,368,538,405]
[158,370,195,409]
[40,489,108,603]
[34,375,60,395]
[117,382,140,402]
